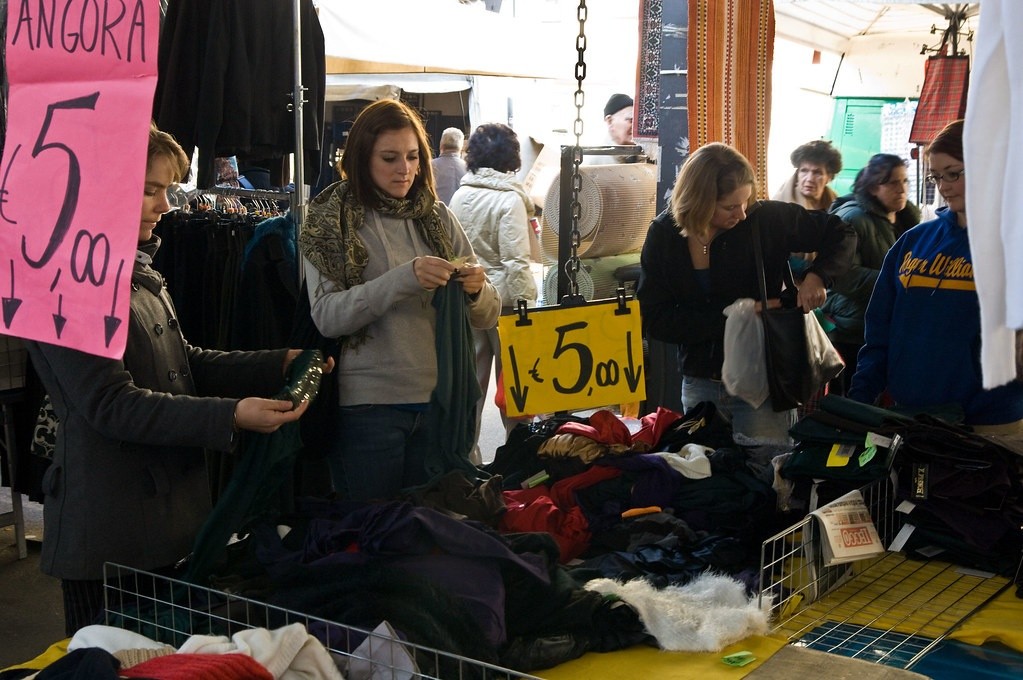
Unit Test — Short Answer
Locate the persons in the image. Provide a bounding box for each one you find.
[821,153,921,395]
[21,127,335,638]
[447,124,537,467]
[588,94,654,165]
[216,157,237,184]
[432,127,469,207]
[848,120,1023,425]
[636,142,846,445]
[771,141,842,419]
[300,100,502,514]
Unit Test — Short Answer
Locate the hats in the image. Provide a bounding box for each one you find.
[604,94,633,119]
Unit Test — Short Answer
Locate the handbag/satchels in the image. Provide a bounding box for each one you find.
[753,199,846,412]
[721,298,767,409]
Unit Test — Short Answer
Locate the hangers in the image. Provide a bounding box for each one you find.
[160,183,318,252]
[505,257,633,330]
[918,18,976,59]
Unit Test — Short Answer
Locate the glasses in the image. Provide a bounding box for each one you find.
[925,169,964,184]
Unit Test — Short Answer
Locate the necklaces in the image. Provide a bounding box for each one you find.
[694,232,718,255]
